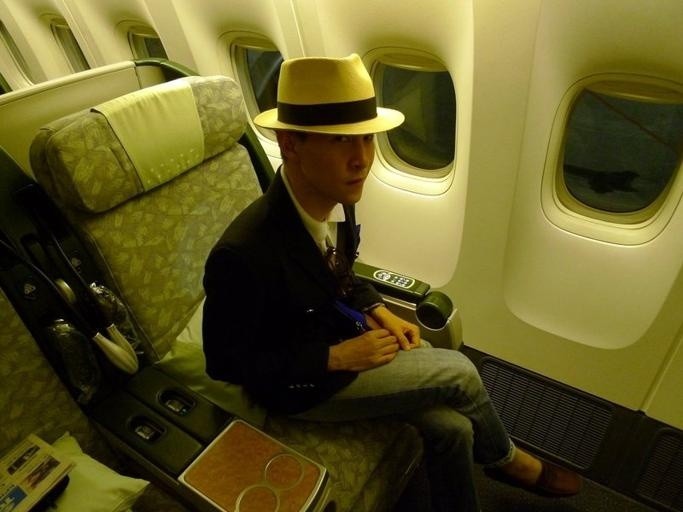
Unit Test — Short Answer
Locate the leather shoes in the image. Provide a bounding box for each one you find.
[485,444,581,497]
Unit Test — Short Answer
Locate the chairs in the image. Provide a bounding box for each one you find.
[28,74,480,512]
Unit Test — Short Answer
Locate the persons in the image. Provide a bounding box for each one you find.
[195,54,586,512]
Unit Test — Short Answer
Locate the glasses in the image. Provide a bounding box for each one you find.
[329,247,356,297]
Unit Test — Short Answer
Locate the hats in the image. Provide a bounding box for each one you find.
[253,52,405,135]
